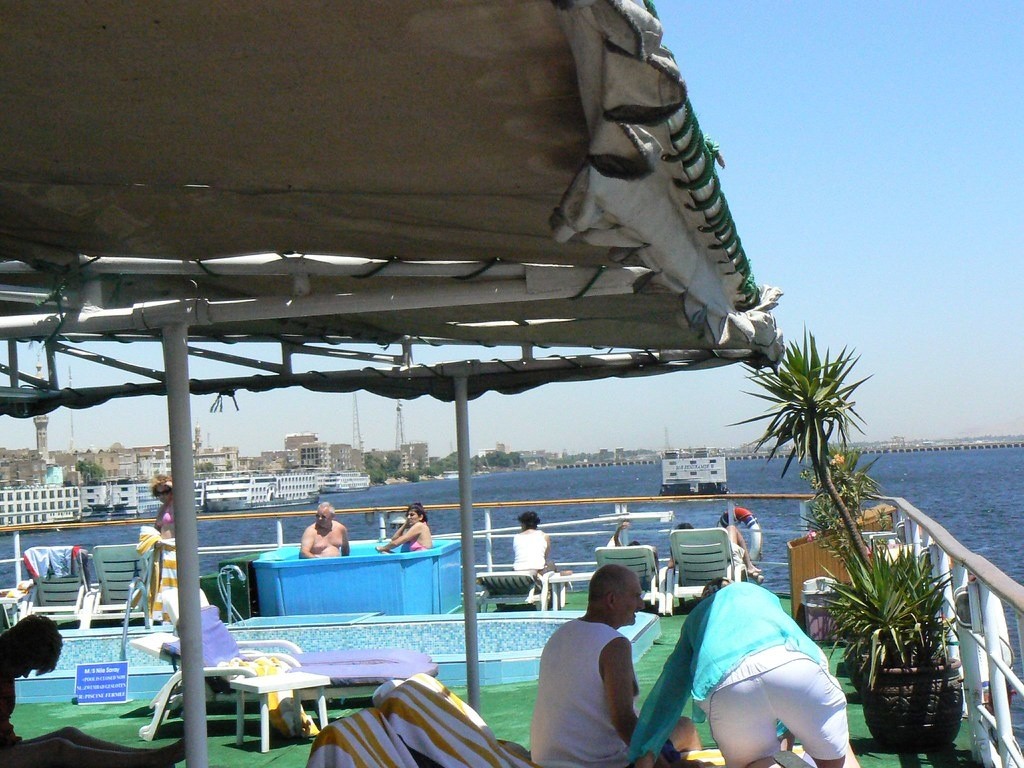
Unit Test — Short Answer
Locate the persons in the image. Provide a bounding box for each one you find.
[513,510,557,574]
[0,615,186,768]
[300,502,350,558]
[627,576,849,768]
[668,523,765,584]
[613,522,639,547]
[376,503,432,554]
[530,564,702,768]
[152,474,176,539]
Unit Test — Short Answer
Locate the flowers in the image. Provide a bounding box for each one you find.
[721,318,966,684]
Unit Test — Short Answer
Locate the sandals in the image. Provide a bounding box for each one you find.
[752,573,764,585]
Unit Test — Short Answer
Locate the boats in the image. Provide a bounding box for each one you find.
[659,448,728,495]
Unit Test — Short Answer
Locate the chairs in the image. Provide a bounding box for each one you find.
[596,544,669,616]
[474,570,566,611]
[129,587,541,768]
[84,544,169,628]
[26,546,99,631]
[666,526,749,616]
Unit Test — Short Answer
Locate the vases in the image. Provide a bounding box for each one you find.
[840,643,881,695]
[861,656,964,747]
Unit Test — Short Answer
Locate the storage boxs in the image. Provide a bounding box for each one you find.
[800,590,841,606]
[786,503,898,635]
[802,602,844,640]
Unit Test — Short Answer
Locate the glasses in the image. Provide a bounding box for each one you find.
[157,489,172,497]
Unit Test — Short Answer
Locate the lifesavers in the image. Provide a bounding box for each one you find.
[716,507,763,560]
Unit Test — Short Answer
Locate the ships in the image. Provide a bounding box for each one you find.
[205,470,321,511]
[321,468,371,493]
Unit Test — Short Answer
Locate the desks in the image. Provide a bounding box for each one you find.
[0,588,26,625]
[549,571,595,611]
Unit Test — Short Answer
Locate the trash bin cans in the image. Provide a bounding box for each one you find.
[800,576,844,641]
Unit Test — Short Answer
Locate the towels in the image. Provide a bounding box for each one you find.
[216,657,321,738]
[305,672,543,768]
[136,525,180,626]
